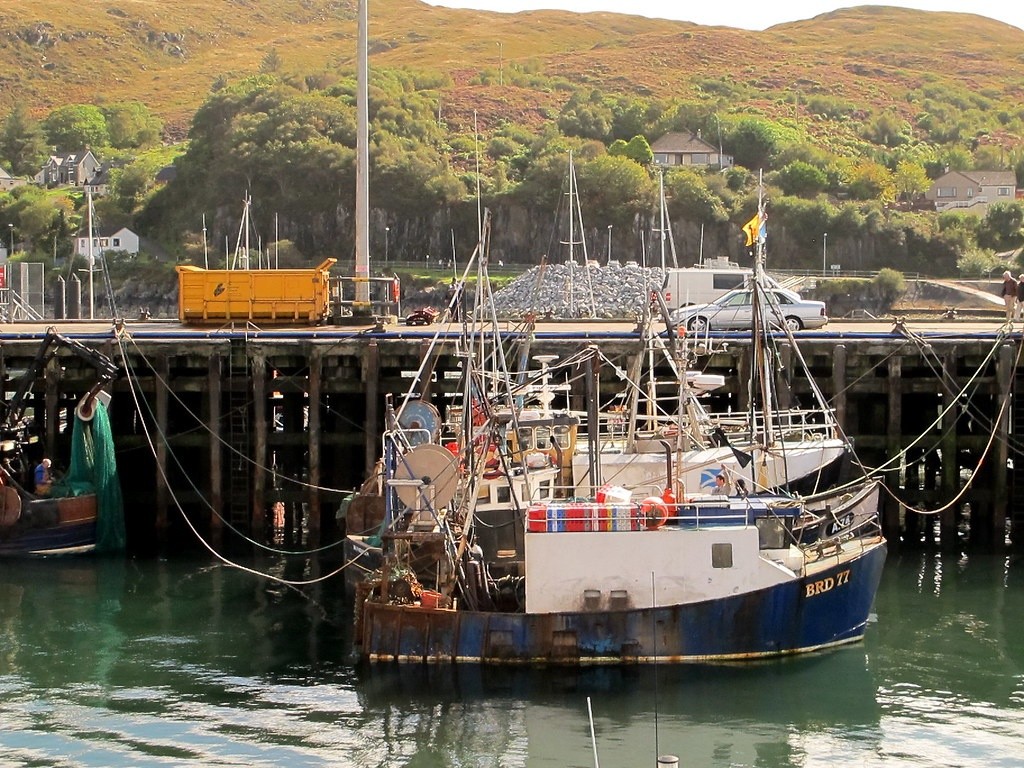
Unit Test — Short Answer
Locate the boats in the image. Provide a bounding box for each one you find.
[0,327,136,555]
[342,109,891,680]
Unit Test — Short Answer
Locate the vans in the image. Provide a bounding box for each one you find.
[658,270,800,320]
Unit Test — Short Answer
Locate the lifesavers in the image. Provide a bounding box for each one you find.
[525,451,558,480]
[475,446,507,480]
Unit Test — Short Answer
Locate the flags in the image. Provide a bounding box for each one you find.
[743,214,760,246]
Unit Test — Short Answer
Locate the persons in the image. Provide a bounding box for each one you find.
[448,277,461,322]
[1001,271,1024,320]
[34,458,56,495]
[712,475,727,495]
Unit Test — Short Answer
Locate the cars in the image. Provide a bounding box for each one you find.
[669,290,828,334]
